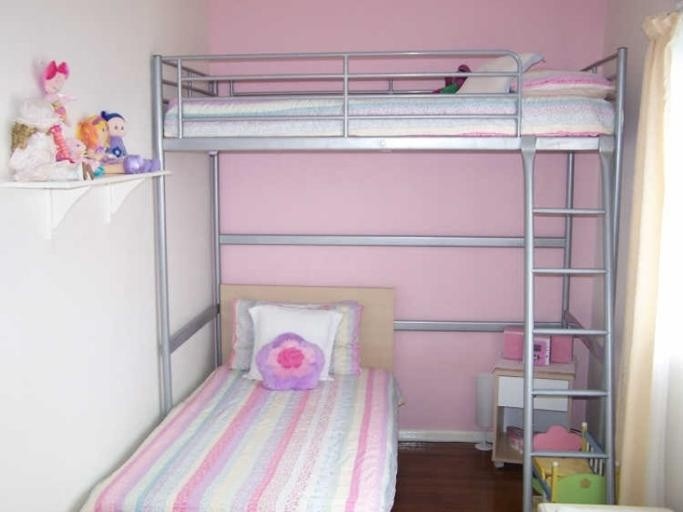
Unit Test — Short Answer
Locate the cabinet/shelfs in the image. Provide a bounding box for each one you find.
[490,362,577,471]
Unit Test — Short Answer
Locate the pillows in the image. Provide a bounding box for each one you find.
[452,53,615,99]
[225,297,362,392]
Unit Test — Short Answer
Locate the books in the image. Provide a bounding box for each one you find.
[525,335,551,366]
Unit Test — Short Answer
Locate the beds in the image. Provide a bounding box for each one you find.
[83,281,403,511]
[150,45,628,156]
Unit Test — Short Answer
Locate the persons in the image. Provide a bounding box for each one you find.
[74,113,144,174]
[100,110,162,174]
[7,99,94,181]
[39,59,70,129]
[47,124,105,178]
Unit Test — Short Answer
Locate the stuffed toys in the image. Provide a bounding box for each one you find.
[432,63,471,95]
